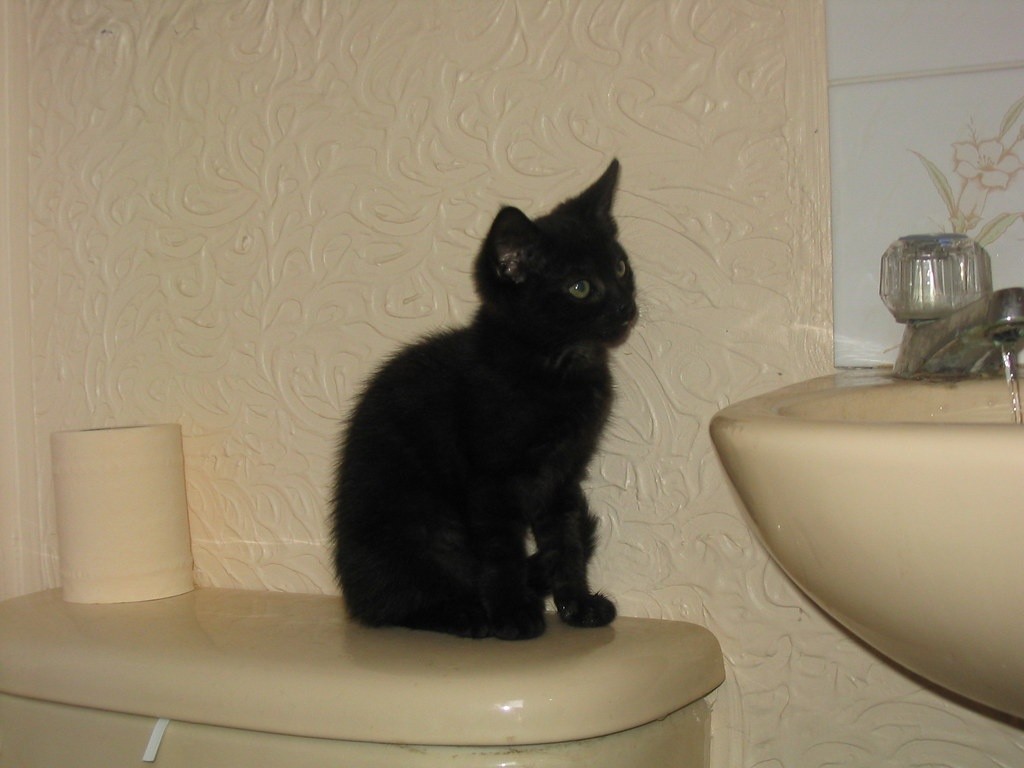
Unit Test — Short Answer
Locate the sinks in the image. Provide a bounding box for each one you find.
[709,368,1024,716]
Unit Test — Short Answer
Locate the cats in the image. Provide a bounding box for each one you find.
[327,156,641,638]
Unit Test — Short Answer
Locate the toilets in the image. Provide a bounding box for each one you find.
[1,586,728,768]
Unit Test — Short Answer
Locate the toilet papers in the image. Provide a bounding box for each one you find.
[49,422,195,605]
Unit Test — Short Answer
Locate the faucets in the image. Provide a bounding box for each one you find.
[877,231,1024,382]
